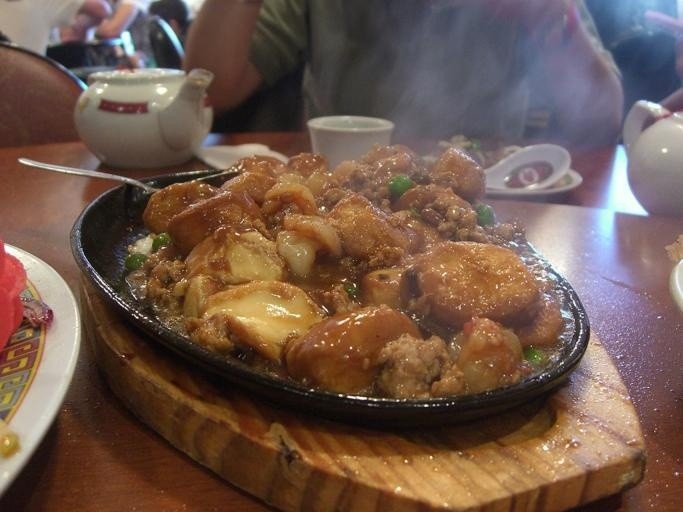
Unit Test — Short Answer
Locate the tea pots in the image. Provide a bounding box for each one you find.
[621,99,683,217]
[72,67,215,171]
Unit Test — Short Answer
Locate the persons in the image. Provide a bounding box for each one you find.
[181,0,628,144]
[43,0,193,68]
[588,0,683,143]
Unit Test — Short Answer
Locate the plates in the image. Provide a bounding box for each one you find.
[485,166,583,196]
[67,167,591,435]
[0,239,83,502]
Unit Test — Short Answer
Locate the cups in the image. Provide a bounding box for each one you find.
[304,114,394,167]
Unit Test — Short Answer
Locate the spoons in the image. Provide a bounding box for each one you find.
[481,143,572,192]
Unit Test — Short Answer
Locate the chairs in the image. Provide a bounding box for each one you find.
[1,41,92,146]
